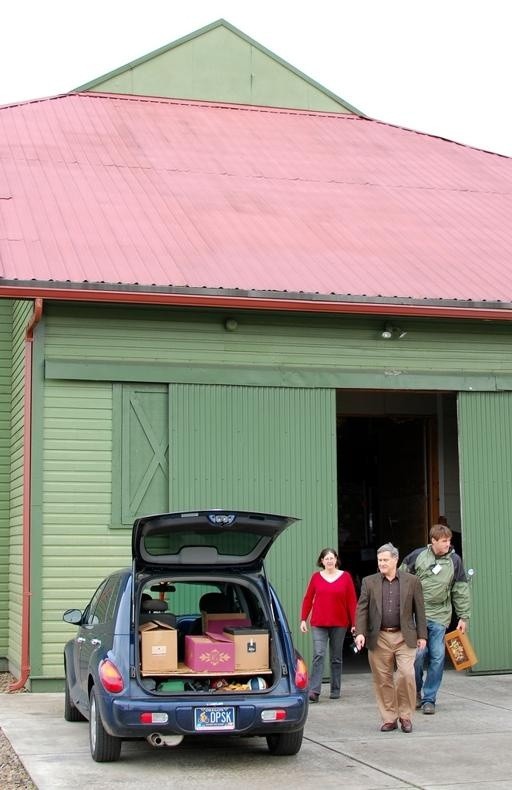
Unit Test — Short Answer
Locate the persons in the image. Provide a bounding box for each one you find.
[300,547,358,700]
[354,541,429,733]
[398,524,472,714]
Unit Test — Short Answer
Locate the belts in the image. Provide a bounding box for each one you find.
[380,626,400,634]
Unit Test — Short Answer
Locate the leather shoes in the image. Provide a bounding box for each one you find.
[399,717,412,734]
[380,721,398,731]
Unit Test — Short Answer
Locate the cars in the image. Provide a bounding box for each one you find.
[62,507,312,762]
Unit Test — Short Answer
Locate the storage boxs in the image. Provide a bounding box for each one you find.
[141,613,269,674]
[444,629,478,671]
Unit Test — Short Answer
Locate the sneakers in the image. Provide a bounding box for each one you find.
[423,702,435,715]
[308,692,320,703]
[415,700,421,709]
[329,689,341,699]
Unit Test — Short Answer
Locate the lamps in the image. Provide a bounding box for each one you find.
[381,320,407,338]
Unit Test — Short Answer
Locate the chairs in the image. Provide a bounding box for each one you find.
[143,598,177,627]
